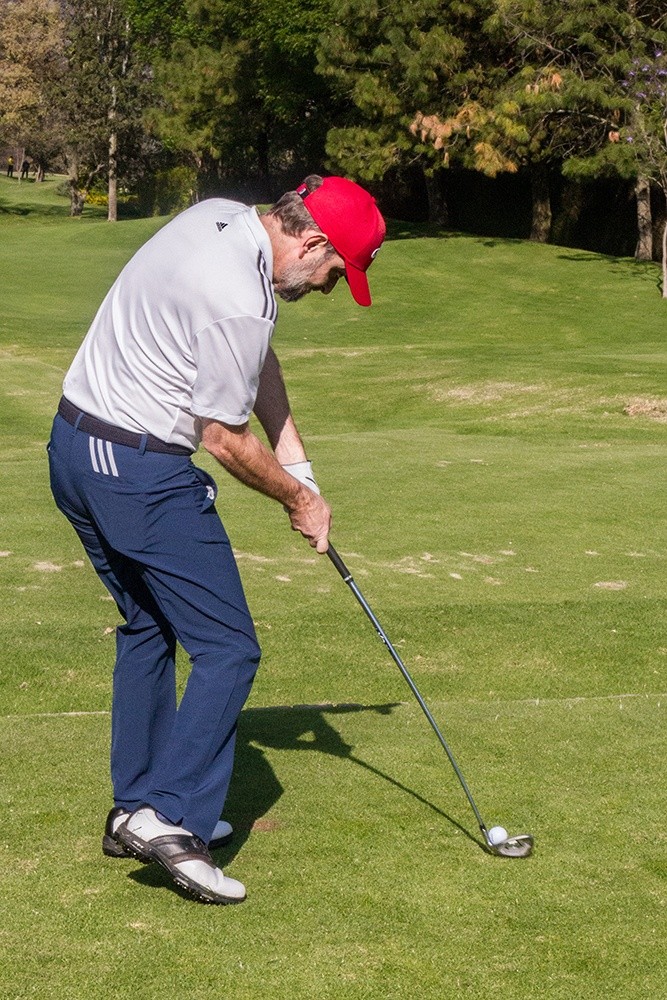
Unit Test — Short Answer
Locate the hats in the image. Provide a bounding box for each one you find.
[296,176,386,306]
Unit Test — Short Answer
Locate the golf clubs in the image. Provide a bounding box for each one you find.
[326,542,536,862]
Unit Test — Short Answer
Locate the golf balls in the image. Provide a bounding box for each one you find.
[488,826,507,846]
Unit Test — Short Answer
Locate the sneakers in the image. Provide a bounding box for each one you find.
[103,807,234,857]
[113,802,247,905]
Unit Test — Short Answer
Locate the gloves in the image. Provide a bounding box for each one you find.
[280,460,320,512]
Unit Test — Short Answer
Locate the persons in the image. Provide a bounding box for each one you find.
[47,175,384,904]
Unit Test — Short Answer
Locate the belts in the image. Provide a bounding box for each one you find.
[57,396,192,456]
